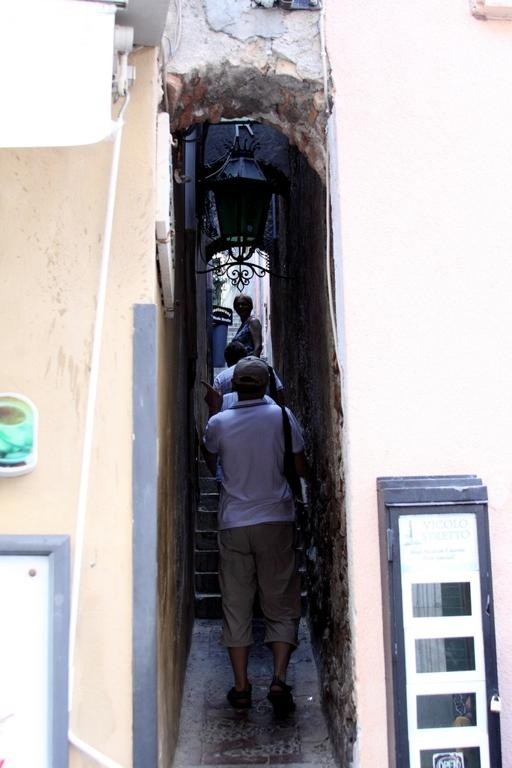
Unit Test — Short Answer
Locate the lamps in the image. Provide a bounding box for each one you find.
[172,117,295,294]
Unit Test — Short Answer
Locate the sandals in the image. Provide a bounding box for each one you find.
[267,678,297,711]
[225,683,253,716]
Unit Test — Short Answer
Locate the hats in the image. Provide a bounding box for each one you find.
[230,354,271,387]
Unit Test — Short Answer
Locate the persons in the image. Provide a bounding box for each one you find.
[212,295,285,493]
[202,356,307,710]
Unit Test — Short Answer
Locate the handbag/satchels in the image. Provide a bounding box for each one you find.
[284,453,308,520]
